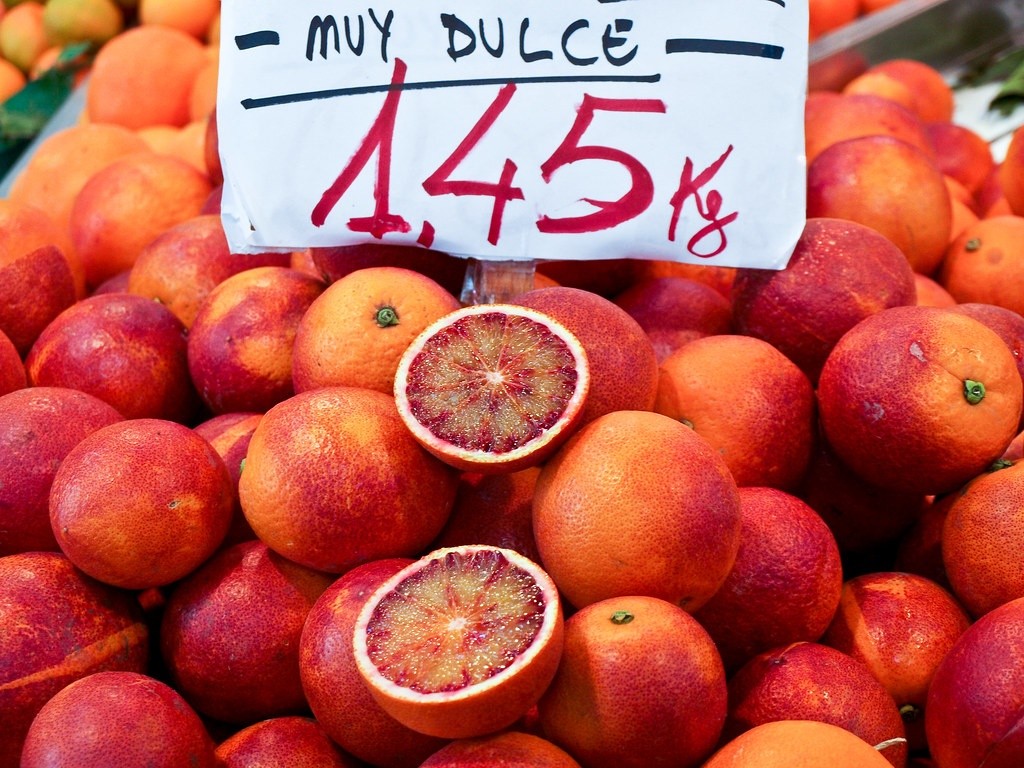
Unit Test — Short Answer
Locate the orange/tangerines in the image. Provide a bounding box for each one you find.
[0,0,1024,768]
